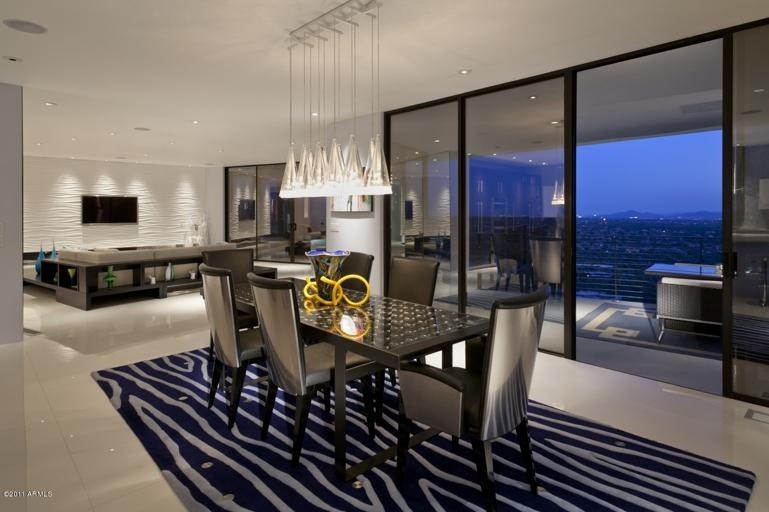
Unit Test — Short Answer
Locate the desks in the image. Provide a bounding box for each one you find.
[645,262,723,279]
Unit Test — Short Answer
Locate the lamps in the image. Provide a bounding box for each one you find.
[277,1,396,207]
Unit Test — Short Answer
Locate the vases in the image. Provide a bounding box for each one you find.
[34,238,57,277]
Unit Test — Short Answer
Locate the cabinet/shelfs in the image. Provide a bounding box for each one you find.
[40,253,203,312]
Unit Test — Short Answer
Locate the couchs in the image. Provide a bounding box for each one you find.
[39,240,237,290]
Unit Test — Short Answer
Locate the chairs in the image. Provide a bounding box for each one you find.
[645,277,726,347]
[192,243,553,510]
[488,226,563,298]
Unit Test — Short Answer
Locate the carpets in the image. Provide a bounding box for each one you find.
[574,302,732,366]
[89,340,760,512]
[733,312,769,366]
[433,283,566,326]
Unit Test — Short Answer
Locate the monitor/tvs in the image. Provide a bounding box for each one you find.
[405,200,412,219]
[238,199,255,220]
[81,194,139,226]
